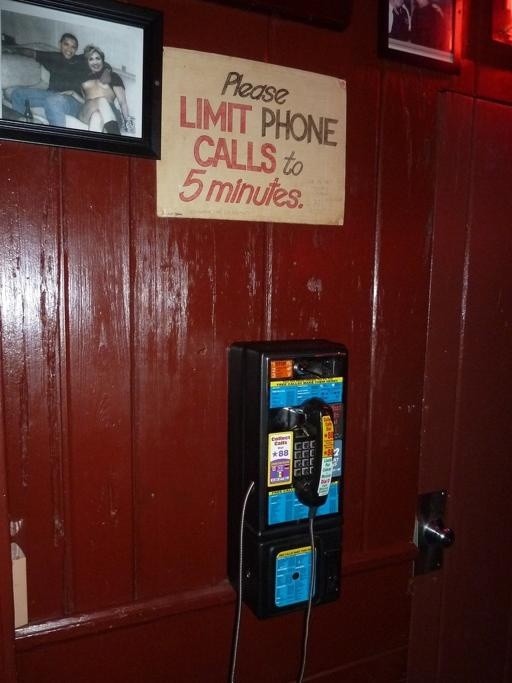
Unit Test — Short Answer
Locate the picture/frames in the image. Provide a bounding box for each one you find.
[377,0,463,74]
[0,0,163,160]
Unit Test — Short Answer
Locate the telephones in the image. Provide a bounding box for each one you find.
[228,338,348,620]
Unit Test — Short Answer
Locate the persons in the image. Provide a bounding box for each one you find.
[58,44,131,136]
[411,0,444,50]
[388,0,411,41]
[2,32,113,127]
